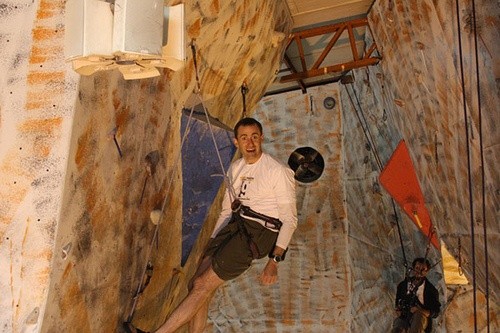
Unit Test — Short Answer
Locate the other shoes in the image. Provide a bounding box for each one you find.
[123,322,150,333]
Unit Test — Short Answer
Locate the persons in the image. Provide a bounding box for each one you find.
[393,257,441,333]
[153,116,299,333]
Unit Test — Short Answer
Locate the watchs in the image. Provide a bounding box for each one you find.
[271,254,281,263]
